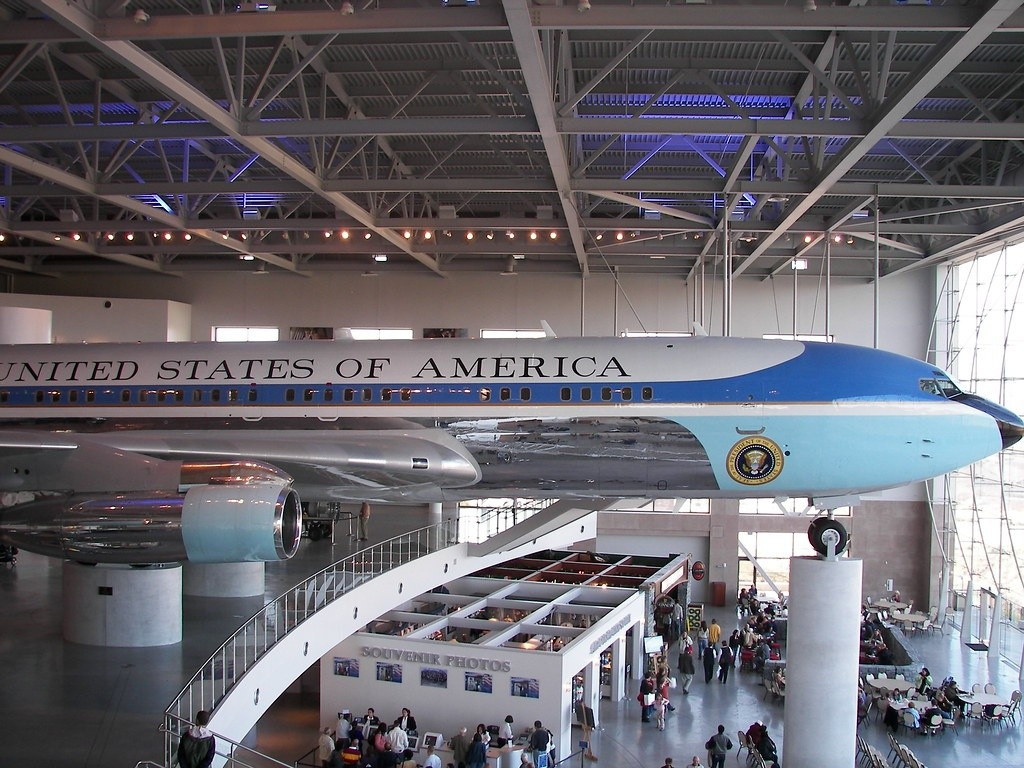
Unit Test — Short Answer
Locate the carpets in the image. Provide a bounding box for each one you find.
[965,642,989,651]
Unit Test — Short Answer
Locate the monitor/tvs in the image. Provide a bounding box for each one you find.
[408,738,417,748]
[425,736,437,746]
[368,728,378,736]
[357,726,363,732]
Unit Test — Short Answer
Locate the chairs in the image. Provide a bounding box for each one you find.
[967,682,1023,730]
[856,732,928,768]
[763,677,785,707]
[858,672,959,738]
[737,731,774,768]
[863,596,954,639]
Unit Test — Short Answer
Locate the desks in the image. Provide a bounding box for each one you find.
[958,693,1007,712]
[889,700,932,714]
[868,679,915,691]
[874,602,907,613]
[892,614,927,627]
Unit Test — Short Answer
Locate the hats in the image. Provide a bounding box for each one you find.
[685,647,688,650]
[755,720,763,726]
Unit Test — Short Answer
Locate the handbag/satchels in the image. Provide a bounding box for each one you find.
[721,653,731,665]
[688,646,693,653]
[729,655,734,664]
[644,693,655,706]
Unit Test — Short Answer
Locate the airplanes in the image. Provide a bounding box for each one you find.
[0,337,1024,566]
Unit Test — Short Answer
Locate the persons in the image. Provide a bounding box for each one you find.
[319,590,970,768]
[359,502,371,540]
[177,710,216,768]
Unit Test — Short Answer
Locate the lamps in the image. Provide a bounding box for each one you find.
[657,233,663,240]
[108,232,115,240]
[443,230,452,237]
[242,231,248,239]
[487,230,493,239]
[127,232,134,240]
[223,231,229,239]
[680,234,687,240]
[847,235,853,243]
